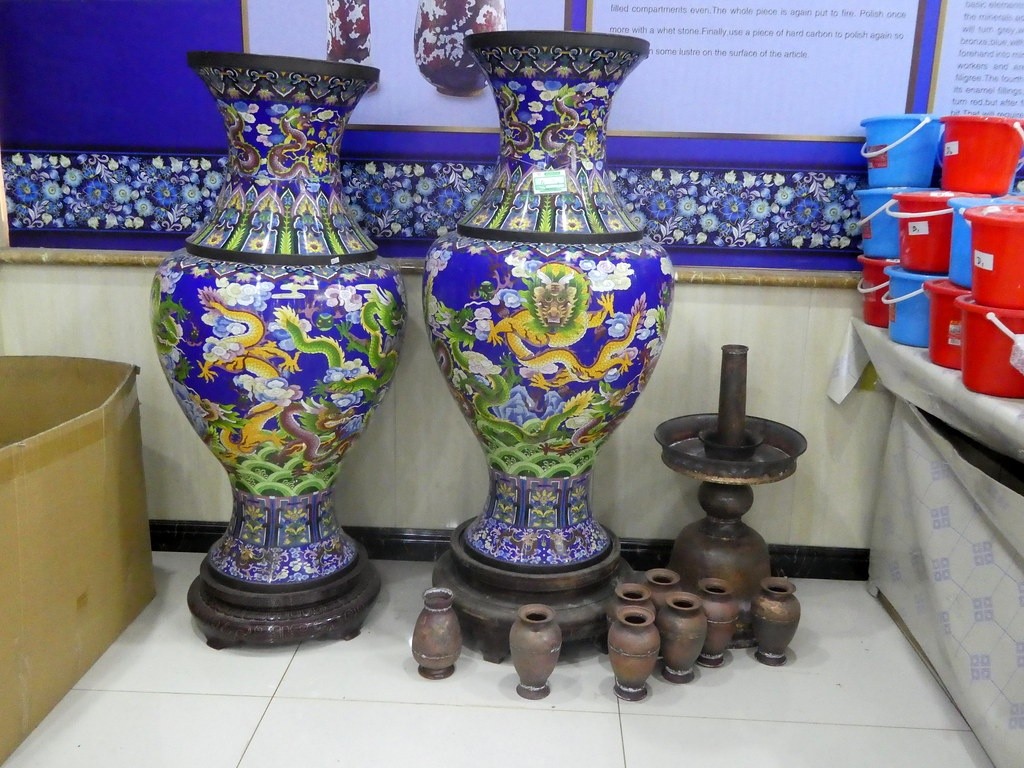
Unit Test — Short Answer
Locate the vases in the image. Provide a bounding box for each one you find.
[695,577,739,668]
[508,602,562,700]
[148,49,411,591]
[749,577,802,666]
[421,28,679,570]
[656,592,708,685]
[643,568,683,609]
[412,586,463,680]
[607,583,656,629]
[607,606,661,702]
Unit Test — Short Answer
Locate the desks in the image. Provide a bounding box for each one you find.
[855,321,1024,768]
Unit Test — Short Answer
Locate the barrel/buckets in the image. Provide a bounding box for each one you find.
[940,116,1024,195]
[882,265,949,347]
[858,255,898,327]
[853,187,940,259]
[964,205,1024,310]
[860,114,939,187]
[947,197,1024,288]
[955,294,1024,398]
[924,280,972,369]
[887,192,982,272]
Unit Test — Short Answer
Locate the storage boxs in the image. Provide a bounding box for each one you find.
[0,355,158,768]
